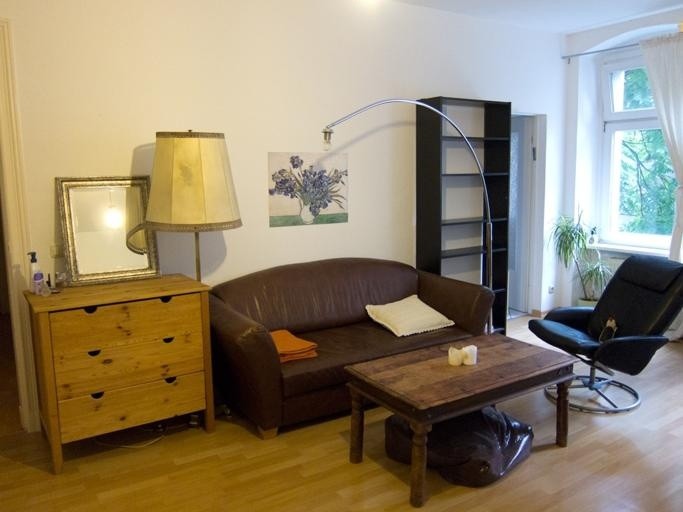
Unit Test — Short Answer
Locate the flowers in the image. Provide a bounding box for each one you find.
[268,153,347,219]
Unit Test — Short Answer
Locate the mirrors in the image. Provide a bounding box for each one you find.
[53,176,160,287]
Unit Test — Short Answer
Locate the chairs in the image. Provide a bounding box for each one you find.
[527,254,682,413]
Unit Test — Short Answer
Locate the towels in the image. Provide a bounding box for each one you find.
[269,329,318,363]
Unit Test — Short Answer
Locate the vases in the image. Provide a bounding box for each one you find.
[299,199,315,225]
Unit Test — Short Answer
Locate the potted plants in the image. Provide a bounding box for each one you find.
[551,204,614,307]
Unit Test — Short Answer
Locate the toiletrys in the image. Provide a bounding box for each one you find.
[27,251,64,298]
[27,251,39,293]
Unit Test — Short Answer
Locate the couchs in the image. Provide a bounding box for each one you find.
[207,255,497,437]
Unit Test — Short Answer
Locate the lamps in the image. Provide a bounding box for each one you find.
[319,98,497,336]
[147,128,242,283]
[123,188,147,255]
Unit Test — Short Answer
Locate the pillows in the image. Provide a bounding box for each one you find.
[364,293,455,339]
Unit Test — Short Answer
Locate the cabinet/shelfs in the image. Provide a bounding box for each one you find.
[21,273,216,476]
[415,95,512,335]
